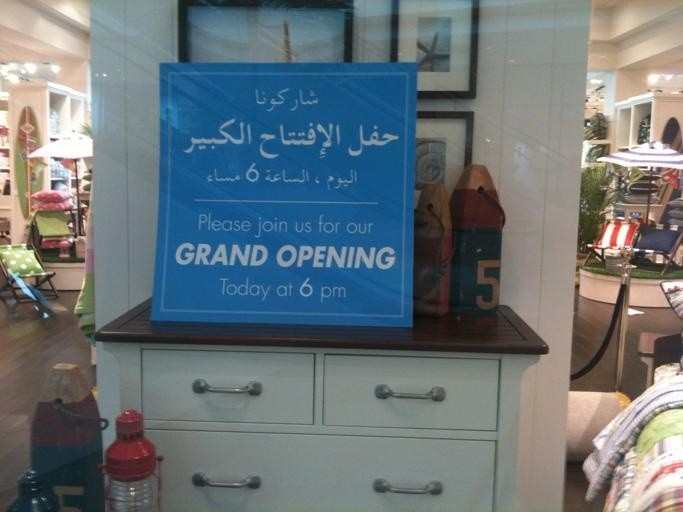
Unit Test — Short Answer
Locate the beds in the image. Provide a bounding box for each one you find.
[580,333,683,511]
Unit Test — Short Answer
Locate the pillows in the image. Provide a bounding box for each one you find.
[667,198,683,225]
[620,170,661,204]
[31,190,74,211]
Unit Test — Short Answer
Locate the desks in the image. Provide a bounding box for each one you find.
[637,332,668,391]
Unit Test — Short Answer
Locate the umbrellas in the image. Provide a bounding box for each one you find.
[27,129,92,235]
[597,139,683,238]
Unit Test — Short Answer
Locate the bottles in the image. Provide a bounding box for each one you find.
[9,470,60,512]
[104,408,158,512]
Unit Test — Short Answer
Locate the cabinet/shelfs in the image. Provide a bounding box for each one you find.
[614,93,683,153]
[0,96,12,240]
[94,297,548,511]
[10,83,90,245]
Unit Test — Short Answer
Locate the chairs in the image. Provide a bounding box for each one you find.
[626,224,683,276]
[32,209,75,258]
[0,242,59,304]
[616,182,674,223]
[659,280,682,320]
[583,220,642,266]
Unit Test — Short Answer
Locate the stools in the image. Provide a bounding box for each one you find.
[566,391,630,488]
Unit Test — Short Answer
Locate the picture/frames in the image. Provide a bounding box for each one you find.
[391,0,480,100]
[410,110,474,210]
[178,0,353,63]
